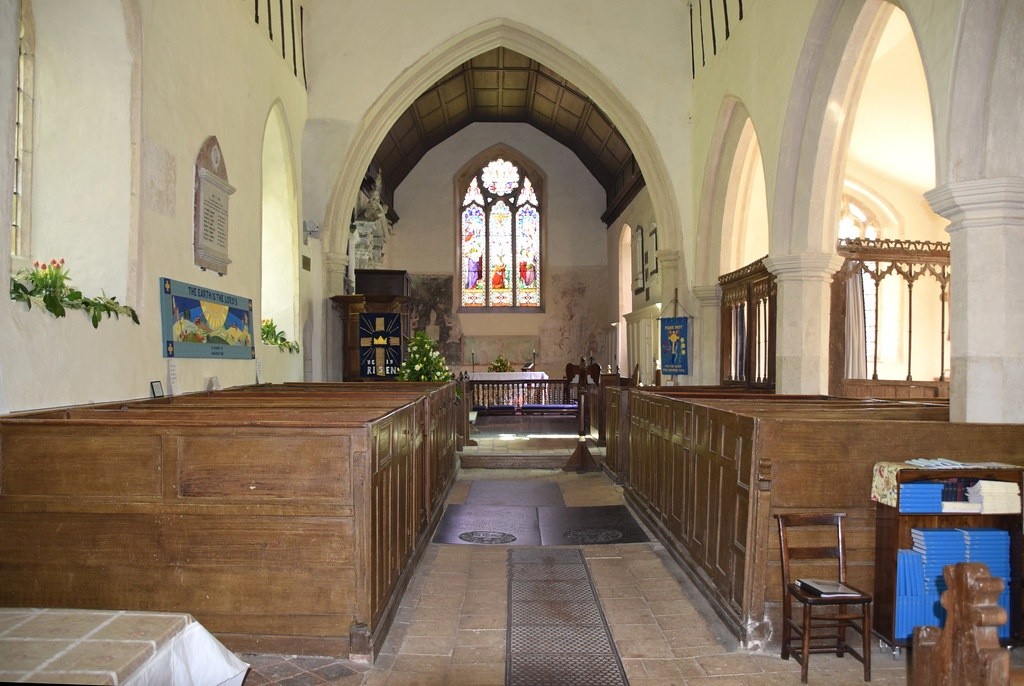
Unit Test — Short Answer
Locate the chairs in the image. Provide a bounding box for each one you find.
[771,512,873,684]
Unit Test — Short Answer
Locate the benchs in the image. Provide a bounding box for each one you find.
[521,404,579,416]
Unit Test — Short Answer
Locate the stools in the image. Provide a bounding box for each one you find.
[485,404,516,415]
[472,406,487,416]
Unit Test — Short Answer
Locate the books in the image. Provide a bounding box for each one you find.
[896,477,1022,639]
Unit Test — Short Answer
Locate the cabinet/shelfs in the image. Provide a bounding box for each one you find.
[869,462,1024,660]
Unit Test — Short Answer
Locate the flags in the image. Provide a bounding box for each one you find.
[661,317,688,375]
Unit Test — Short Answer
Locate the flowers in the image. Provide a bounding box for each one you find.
[487,353,515,373]
[260,318,301,355]
[396,328,462,398]
[9,259,141,331]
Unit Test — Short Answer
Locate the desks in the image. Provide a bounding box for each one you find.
[455,371,549,410]
